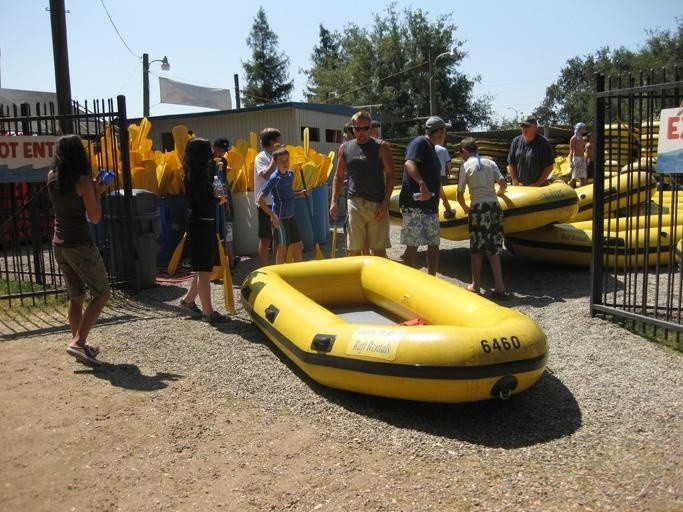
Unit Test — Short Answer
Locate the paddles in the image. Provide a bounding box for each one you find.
[86,117,187,195]
[225,132,256,193]
[215,200,236,314]
[283,127,335,191]
[166,228,191,274]
[300,168,323,258]
[330,195,337,258]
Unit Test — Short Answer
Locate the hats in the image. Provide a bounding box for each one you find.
[425,116,452,135]
[518,116,537,126]
[215,137,229,152]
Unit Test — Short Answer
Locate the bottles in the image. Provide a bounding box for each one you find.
[413,192,436,201]
[212,176,225,199]
[95,168,115,188]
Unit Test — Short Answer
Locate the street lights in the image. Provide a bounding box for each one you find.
[143,52,169,117]
[426,50,457,115]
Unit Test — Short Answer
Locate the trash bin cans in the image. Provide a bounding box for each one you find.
[103,189,160,289]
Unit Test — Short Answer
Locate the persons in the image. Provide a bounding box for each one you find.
[508,116,555,187]
[567,122,594,188]
[179,129,240,322]
[47,134,111,366]
[457,137,507,298]
[329,111,394,261]
[254,127,307,267]
[400,116,453,277]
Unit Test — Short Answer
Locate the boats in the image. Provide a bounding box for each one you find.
[241,256,548,404]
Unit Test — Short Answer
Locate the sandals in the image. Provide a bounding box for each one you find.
[67,344,103,367]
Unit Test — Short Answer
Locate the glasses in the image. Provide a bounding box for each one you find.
[354,126,370,131]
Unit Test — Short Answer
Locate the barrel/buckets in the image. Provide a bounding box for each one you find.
[232,190,260,256]
[152,194,187,273]
[292,191,314,250]
[312,182,330,244]
[105,210,161,290]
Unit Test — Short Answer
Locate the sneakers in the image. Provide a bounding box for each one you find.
[178,298,203,317]
[201,311,231,323]
[487,287,510,300]
